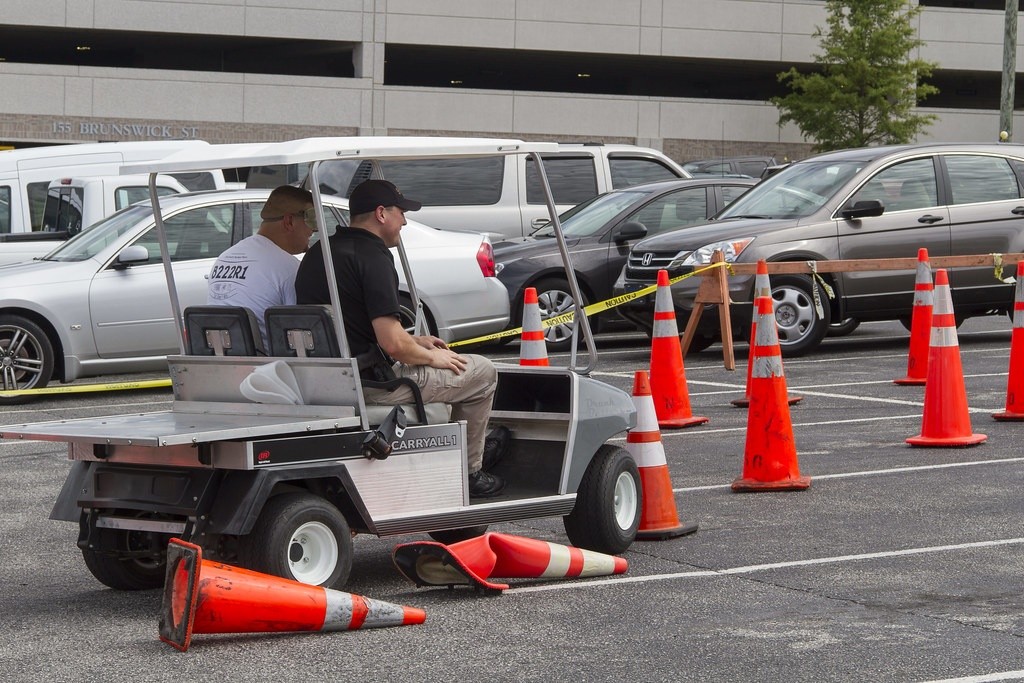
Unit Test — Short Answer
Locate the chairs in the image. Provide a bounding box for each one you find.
[860,180,930,211]
[674,195,701,224]
[183,303,453,427]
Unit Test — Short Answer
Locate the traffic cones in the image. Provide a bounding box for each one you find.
[649,269,709,428]
[893,247,935,385]
[625,371,699,540]
[730,258,804,407]
[392,533,629,597]
[157,538,426,654]
[905,269,989,448]
[518,287,550,366]
[990,261,1024,422]
[731,296,811,491]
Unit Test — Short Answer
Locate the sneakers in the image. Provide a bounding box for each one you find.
[468,470,506,497]
[482,424,511,472]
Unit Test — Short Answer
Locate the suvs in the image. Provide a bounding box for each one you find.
[611,142,1024,359]
[681,154,775,179]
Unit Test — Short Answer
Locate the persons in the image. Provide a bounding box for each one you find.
[201,183,321,359]
[297,180,508,498]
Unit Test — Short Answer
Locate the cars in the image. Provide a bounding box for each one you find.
[493,176,828,353]
[0,188,512,408]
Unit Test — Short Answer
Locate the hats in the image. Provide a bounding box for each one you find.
[349,179,421,215]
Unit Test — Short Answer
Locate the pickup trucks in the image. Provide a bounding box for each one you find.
[0,140,251,267]
[287,137,731,236]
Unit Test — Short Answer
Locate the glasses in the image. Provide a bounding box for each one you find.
[302,207,319,231]
